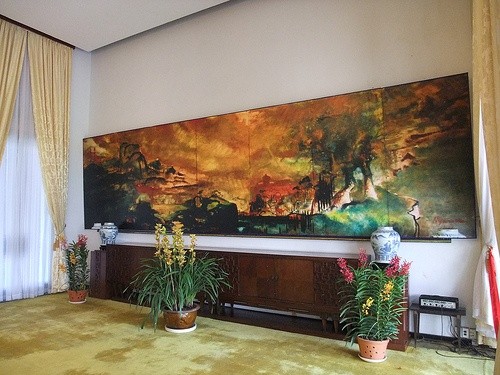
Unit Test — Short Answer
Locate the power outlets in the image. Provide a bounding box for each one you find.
[463,328,470,338]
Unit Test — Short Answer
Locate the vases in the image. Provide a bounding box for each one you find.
[370,227,401,261]
[99,223,118,245]
[357,334,389,359]
[162,304,198,329]
[68,288,88,302]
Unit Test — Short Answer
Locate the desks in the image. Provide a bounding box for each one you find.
[380,271,410,352]
[90,250,114,300]
[106,244,371,341]
[409,303,466,354]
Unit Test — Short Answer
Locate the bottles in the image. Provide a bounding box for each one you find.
[370,226,403,264]
[99,222,118,246]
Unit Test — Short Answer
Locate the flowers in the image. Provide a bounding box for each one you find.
[122,220,233,333]
[56,234,91,292]
[336,248,414,348]
